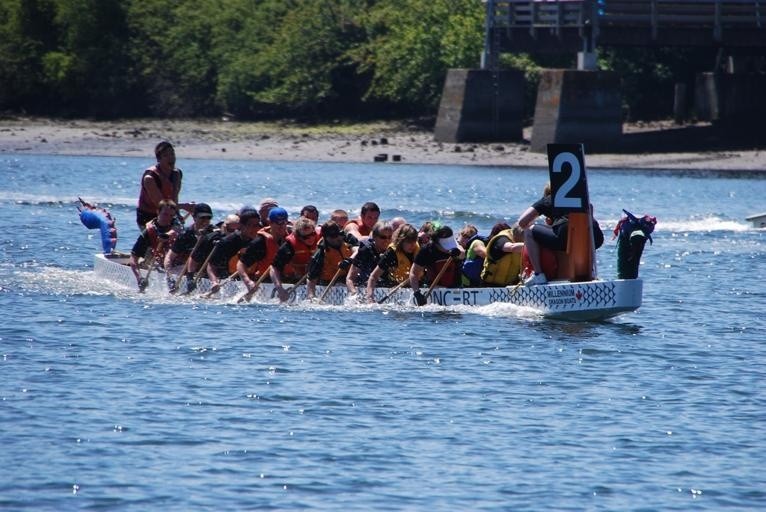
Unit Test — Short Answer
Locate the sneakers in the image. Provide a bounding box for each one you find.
[523,270,548,287]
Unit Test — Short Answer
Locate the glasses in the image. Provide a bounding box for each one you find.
[226,224,241,233]
[296,230,317,240]
[243,221,261,228]
[272,219,291,226]
[324,229,346,239]
[374,231,393,240]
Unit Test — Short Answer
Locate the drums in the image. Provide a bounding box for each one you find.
[521,239,560,281]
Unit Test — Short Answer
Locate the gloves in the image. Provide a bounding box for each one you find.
[137,276,149,292]
[447,248,461,258]
[184,271,198,292]
[411,289,428,307]
[165,276,179,294]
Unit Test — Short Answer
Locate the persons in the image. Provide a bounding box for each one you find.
[216,206,257,229]
[136,141,182,268]
[207,208,264,292]
[238,208,294,293]
[410,227,465,305]
[331,210,347,229]
[345,223,394,297]
[341,202,380,245]
[364,224,426,305]
[300,206,318,226]
[457,224,512,287]
[304,220,360,303]
[127,198,185,292]
[184,214,240,290]
[258,198,293,228]
[389,217,406,233]
[517,182,604,286]
[166,203,215,293]
[267,217,322,302]
[417,222,436,249]
[453,227,478,287]
[479,221,534,287]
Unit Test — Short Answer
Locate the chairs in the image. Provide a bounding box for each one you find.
[539,213,593,281]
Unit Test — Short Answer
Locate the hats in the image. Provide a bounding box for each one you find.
[435,226,459,251]
[191,203,214,219]
[268,207,288,222]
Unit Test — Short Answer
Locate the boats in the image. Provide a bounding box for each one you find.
[745,213,766,228]
[75,197,657,323]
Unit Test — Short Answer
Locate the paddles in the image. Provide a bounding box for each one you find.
[238,267,272,302]
